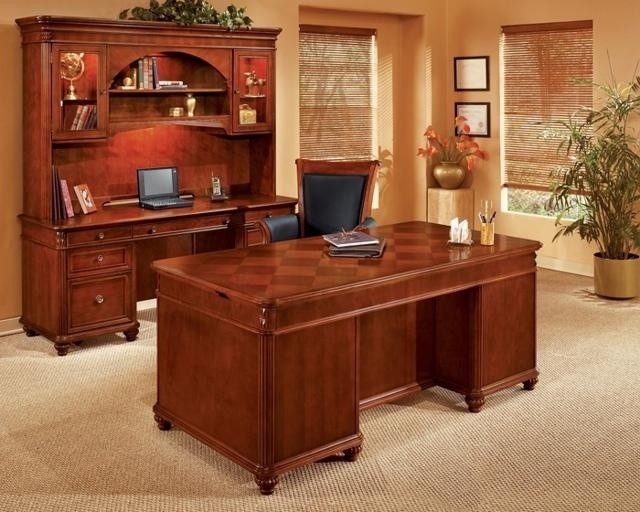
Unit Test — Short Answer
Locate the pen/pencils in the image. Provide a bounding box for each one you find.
[478,212,497,224]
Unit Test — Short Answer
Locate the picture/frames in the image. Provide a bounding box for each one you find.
[454,56,488,91]
[454,102,490,137]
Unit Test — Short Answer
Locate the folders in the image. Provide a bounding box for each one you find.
[328,236,386,257]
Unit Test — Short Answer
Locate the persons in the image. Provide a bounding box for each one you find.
[82,189,92,206]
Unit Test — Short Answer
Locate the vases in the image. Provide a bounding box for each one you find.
[434,163,464,189]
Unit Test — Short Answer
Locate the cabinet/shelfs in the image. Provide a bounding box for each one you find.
[108,89,232,134]
[15,16,107,143]
[232,27,284,136]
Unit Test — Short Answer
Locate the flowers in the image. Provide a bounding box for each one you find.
[417,117,486,170]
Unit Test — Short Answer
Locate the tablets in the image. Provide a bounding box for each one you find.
[322,230,380,248]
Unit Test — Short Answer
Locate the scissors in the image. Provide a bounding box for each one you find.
[480,199,492,218]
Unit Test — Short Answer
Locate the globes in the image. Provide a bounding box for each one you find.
[61,53,85,100]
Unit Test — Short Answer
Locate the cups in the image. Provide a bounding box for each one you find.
[480,222,495,246]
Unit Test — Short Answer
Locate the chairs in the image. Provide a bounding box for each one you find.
[261,159,380,243]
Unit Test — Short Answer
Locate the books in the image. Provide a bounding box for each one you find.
[322,231,385,257]
[64,104,97,130]
[130,56,159,89]
[159,80,188,88]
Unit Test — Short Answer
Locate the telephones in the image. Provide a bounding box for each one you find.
[208,170,229,201]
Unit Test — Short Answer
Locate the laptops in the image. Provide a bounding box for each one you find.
[136,166,194,210]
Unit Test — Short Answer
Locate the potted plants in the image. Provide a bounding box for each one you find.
[534,49,640,299]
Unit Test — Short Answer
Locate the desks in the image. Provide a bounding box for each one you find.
[151,221,542,496]
[19,193,298,354]
[428,188,473,228]
